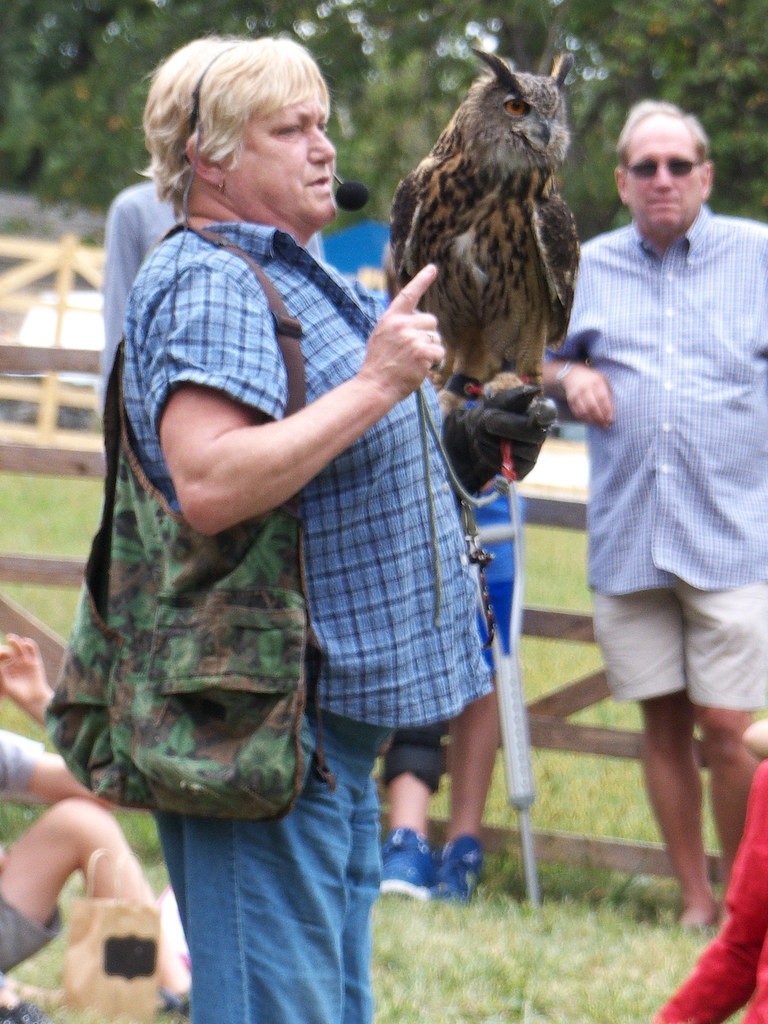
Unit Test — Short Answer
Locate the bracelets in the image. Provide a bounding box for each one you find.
[555,362,572,385]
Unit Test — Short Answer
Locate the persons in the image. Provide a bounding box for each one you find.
[651,720,768,1024]
[537,99,768,931]
[104,34,496,1024]
[0,634,166,1024]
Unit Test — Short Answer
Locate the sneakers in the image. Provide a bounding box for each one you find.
[381,827,438,897]
[438,838,484,900]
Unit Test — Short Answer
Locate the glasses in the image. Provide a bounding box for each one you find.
[624,158,697,179]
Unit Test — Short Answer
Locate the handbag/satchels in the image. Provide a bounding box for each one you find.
[60,844,165,1018]
[42,422,307,825]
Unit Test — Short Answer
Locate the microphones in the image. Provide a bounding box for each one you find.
[333,174,369,212]
